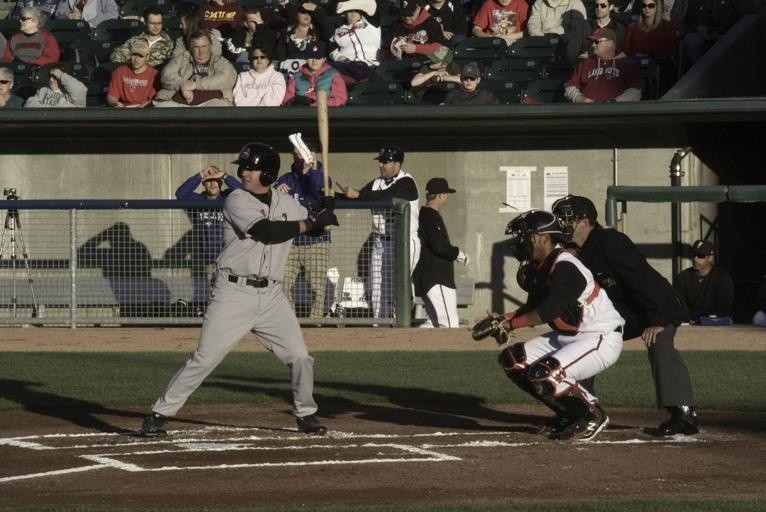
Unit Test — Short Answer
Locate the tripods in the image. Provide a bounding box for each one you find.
[1,210,39,319]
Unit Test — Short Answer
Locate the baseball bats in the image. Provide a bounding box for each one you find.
[318,91,331,231]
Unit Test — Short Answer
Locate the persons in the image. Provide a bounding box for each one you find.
[343,143,422,328]
[274,141,332,328]
[471,209,626,442]
[141,141,339,437]
[174,165,243,317]
[551,194,701,437]
[412,177,470,328]
[672,240,735,326]
[1,1,764,109]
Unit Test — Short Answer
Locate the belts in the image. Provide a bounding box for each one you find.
[227,274,269,288]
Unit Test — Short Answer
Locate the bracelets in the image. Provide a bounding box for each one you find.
[505,312,517,320]
[222,173,227,179]
[511,313,529,328]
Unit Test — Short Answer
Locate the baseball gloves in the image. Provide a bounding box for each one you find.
[473,315,510,345]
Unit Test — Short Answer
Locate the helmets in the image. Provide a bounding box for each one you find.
[506,209,564,263]
[374,144,404,163]
[551,193,598,229]
[230,142,281,173]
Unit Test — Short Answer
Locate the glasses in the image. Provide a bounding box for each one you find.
[593,2,609,10]
[590,39,611,45]
[640,2,656,10]
[0,78,11,84]
[251,55,268,60]
[692,254,709,259]
[22,16,32,22]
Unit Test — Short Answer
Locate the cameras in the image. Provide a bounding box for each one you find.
[5,189,17,196]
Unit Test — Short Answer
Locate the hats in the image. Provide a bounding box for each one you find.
[584,28,617,43]
[304,42,326,60]
[460,63,481,83]
[129,37,150,57]
[690,239,715,257]
[399,0,418,16]
[425,46,454,65]
[424,177,456,195]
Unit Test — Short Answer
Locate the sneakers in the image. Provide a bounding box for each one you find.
[298,415,328,435]
[572,403,611,441]
[141,412,168,437]
[659,404,699,440]
[550,416,585,441]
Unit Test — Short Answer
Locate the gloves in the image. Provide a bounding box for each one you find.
[455,250,471,267]
[303,207,340,237]
